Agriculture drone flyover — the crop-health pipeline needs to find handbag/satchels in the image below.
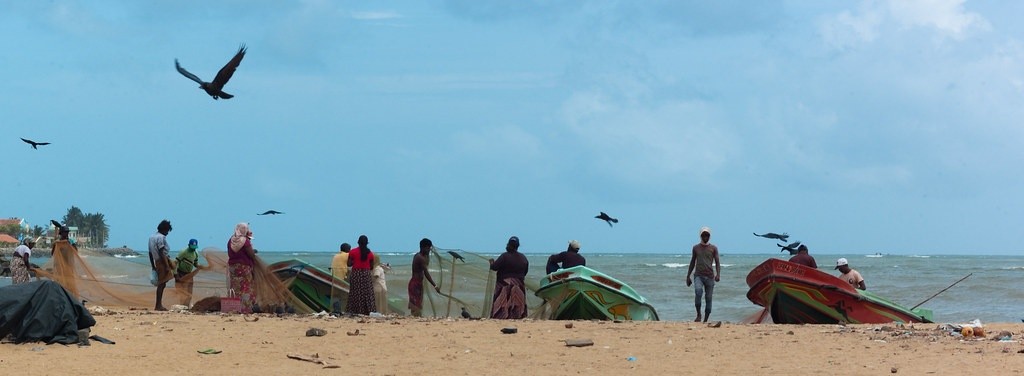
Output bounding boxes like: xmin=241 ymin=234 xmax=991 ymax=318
xmin=168 ymin=257 xmax=176 ymax=269
xmin=220 ymin=289 xmax=240 ymax=313
xmin=151 ymin=270 xmax=158 ymax=285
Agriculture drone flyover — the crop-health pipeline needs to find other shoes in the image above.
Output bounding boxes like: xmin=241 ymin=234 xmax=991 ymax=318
xmin=694 ymin=314 xmax=702 ymax=322
xmin=703 ymin=317 xmax=709 ymax=322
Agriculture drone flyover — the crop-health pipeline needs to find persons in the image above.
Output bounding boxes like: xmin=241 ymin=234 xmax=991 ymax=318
xmin=173 ymin=239 xmax=204 ymax=308
xmin=489 ymin=236 xmax=529 ymax=319
xmin=542 ymin=240 xmax=586 ymax=307
xmin=834 ymin=258 xmax=866 ymax=291
xmin=686 ymin=227 xmax=720 ymax=322
xmin=227 ymin=222 xmax=260 ymax=314
xmin=9 ymin=236 xmax=36 ymax=285
xmin=371 ymin=254 xmax=388 ymax=315
xmin=789 ymin=244 xmax=817 ymax=269
xmin=344 ymin=234 xmax=377 ymax=316
xmin=148 ymin=220 xmax=177 ymax=311
xmin=52 ymin=226 xmax=79 ymax=295
xmin=330 ymin=243 xmax=351 ymax=313
xmin=407 ymin=238 xmax=440 ymax=316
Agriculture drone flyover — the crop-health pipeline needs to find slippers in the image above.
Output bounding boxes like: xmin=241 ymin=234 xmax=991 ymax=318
xmin=155 ymin=306 xmax=168 ymax=311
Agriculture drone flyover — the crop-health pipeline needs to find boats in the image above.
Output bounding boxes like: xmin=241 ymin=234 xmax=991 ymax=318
xmin=746 ymin=259 xmax=936 ymax=325
xmin=268 ymin=260 xmax=409 ymax=315
xmin=534 ymin=265 xmax=659 ymax=321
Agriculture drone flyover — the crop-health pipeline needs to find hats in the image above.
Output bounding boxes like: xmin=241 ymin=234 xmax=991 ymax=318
xmin=568 ymin=239 xmax=580 ymax=251
xmin=247 ymin=231 xmax=254 ymax=240
xmin=58 ymin=226 xmax=71 ymax=234
xmin=834 ymin=258 xmax=848 ymax=270
xmin=188 ymin=239 xmax=198 ymax=249
xmin=22 ymin=236 xmax=37 ymax=244
xmin=508 ymin=236 xmax=519 ymax=245
xmin=700 ymin=227 xmax=711 ymax=237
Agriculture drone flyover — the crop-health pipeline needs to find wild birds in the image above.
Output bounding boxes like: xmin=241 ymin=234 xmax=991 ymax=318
xmin=174 ymin=42 xmax=249 ymax=100
xmin=753 ymin=231 xmax=801 ymax=255
xmin=446 ymin=251 xmax=466 ymax=264
xmin=461 ymin=307 xmax=470 ymax=318
xmin=595 ymin=211 xmax=618 ymax=228
xmin=20 ymin=137 xmax=52 ymax=151
xmin=920 ymin=315 xmax=934 ymax=323
xmin=256 ymin=210 xmax=285 ymax=215
